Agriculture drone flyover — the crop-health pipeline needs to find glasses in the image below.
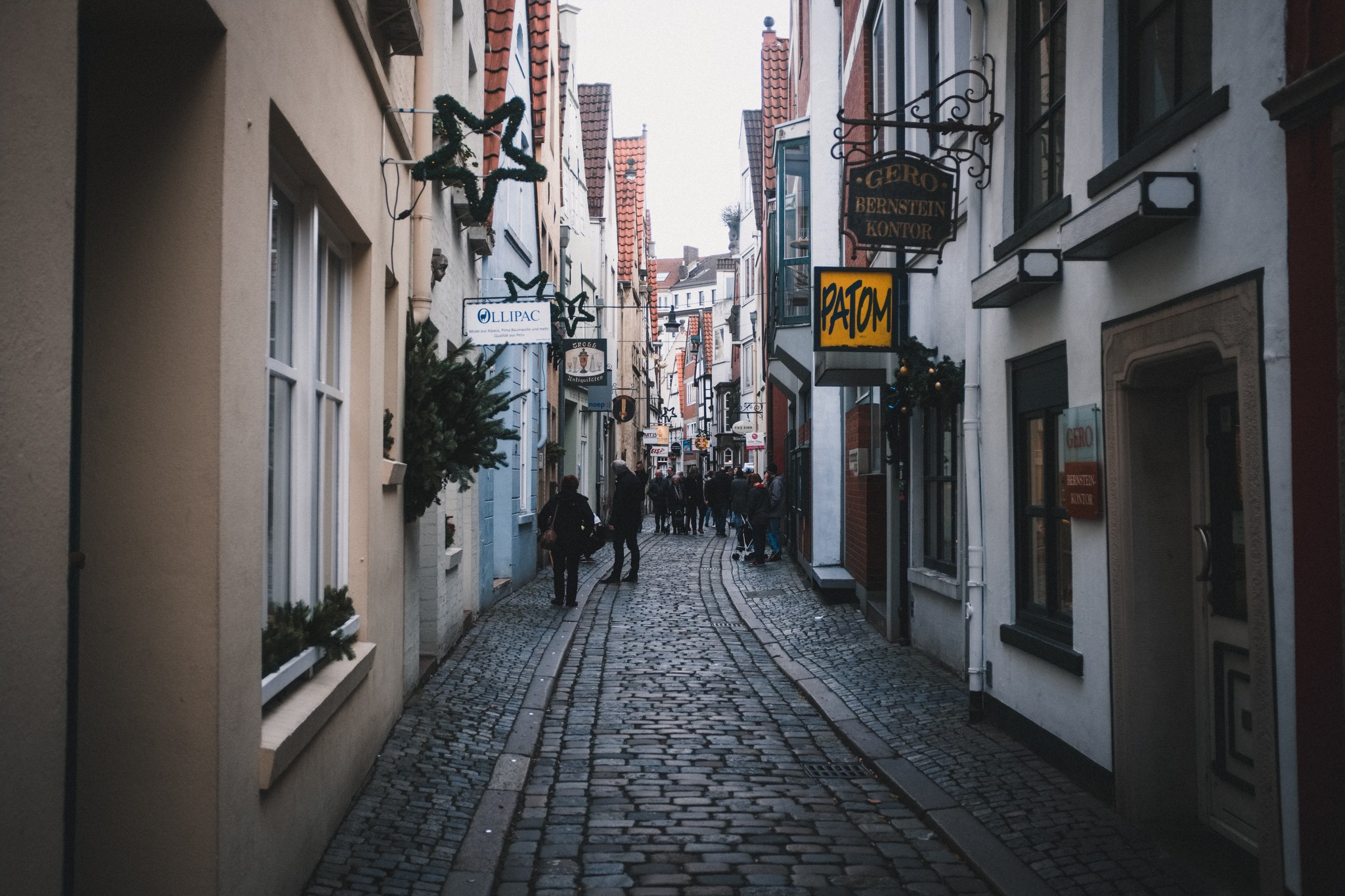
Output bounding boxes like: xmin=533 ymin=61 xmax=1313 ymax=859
xmin=667 ymin=470 xmax=672 ymax=471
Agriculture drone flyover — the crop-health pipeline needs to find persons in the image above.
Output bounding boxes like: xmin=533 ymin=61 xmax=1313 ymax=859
xmin=686 ymin=466 xmax=705 ymax=535
xmin=665 ymin=464 xmax=752 ymax=526
xmin=666 ymin=475 xmax=689 ymax=534
xmin=766 ymin=463 xmax=788 ymax=562
xmin=731 ymin=469 xmax=749 ymax=534
xmin=647 ymin=469 xmax=671 ymax=532
xmin=539 ymin=475 xmax=594 ymax=606
xmin=707 ymin=466 xmax=731 ymax=537
xmin=631 ymin=461 xmax=649 ymax=532
xmin=598 ymin=459 xmax=644 ymax=584
xmin=580 ymin=513 xmax=602 ymax=562
xmin=748 ymin=473 xmax=771 ymax=566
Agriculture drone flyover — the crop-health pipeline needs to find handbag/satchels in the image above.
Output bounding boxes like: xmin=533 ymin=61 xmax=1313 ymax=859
xmin=537 ymin=527 xmax=558 ymax=550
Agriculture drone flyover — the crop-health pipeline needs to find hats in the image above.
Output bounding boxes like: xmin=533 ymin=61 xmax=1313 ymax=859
xmin=768 ymin=464 xmax=778 ymax=474
xmin=748 ymin=473 xmax=763 ymax=486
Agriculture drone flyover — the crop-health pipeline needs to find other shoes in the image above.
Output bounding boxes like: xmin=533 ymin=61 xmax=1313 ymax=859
xmin=622 ymin=575 xmax=638 ymax=581
xmin=566 ymin=601 xmax=578 ymax=606
xmin=636 ymin=512 xmax=741 ymax=537
xmin=748 ymin=561 xmax=765 ymax=567
xmin=599 ymin=574 xmax=620 ymax=584
xmin=769 ymin=550 xmax=781 ymax=562
xmin=578 ymin=557 xmax=596 ymax=562
xmin=551 ymin=599 xmax=563 ymax=605
